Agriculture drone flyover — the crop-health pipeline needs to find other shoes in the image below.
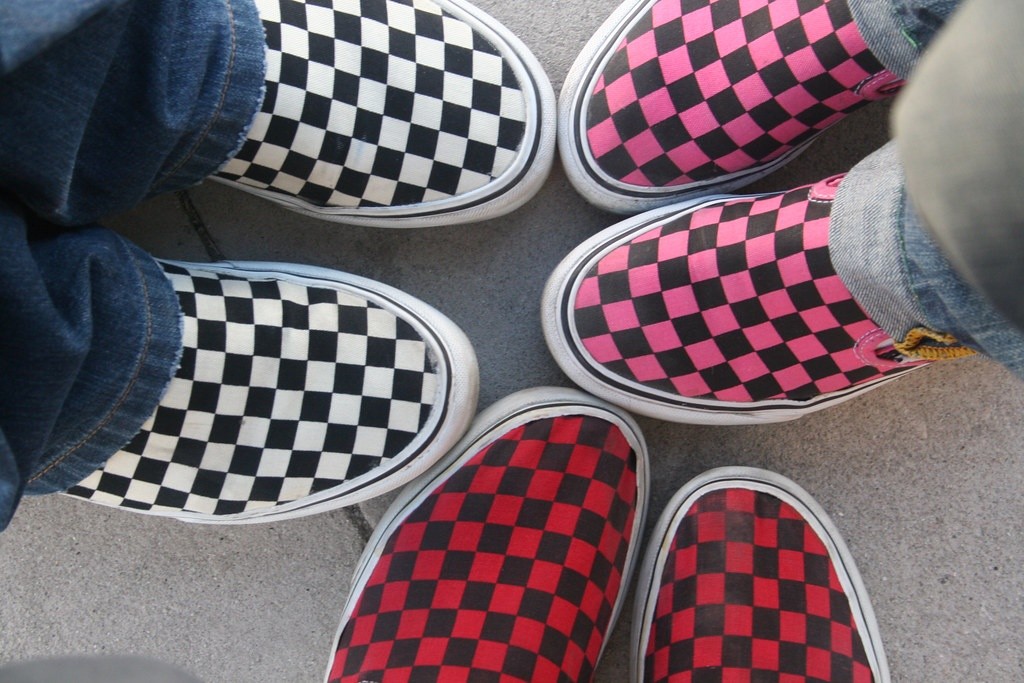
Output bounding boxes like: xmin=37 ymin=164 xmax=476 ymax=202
xmin=628 ymin=467 xmax=891 ymax=683
xmin=556 ymin=0 xmax=906 ymax=215
xmin=203 ymin=0 xmax=556 ymax=229
xmin=52 ymin=260 xmax=480 ymax=524
xmin=539 ymin=172 xmax=941 ymax=426
xmin=319 ymin=386 xmax=651 ymax=683
